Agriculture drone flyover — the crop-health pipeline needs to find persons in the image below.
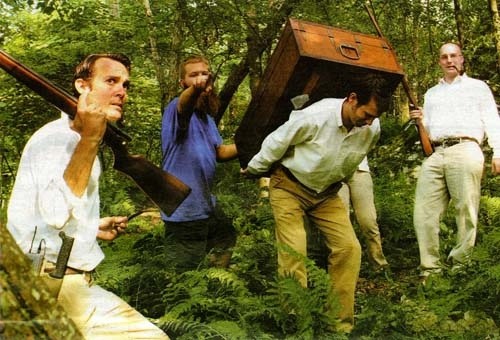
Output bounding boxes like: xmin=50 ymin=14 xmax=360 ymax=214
xmin=337 ymin=157 xmax=387 ymax=271
xmin=240 ymin=75 xmax=381 ymax=340
xmin=161 ymin=57 xmax=239 ymax=275
xmin=409 ymin=43 xmax=500 ymax=291
xmin=7 ymin=53 xmax=168 ymax=339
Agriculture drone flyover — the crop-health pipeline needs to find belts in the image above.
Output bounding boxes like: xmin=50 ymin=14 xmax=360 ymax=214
xmin=44 ymin=267 xmax=82 ymax=275
xmin=433 ymin=138 xmax=466 ymax=146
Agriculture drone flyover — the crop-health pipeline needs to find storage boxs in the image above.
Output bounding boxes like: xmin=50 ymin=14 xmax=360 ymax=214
xmin=232 ymin=17 xmax=406 ymax=178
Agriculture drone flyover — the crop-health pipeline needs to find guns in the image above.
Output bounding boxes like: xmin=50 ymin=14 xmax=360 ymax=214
xmin=0 ymin=50 xmax=191 ymax=218
xmin=363 ymin=1 xmax=434 ymax=157
